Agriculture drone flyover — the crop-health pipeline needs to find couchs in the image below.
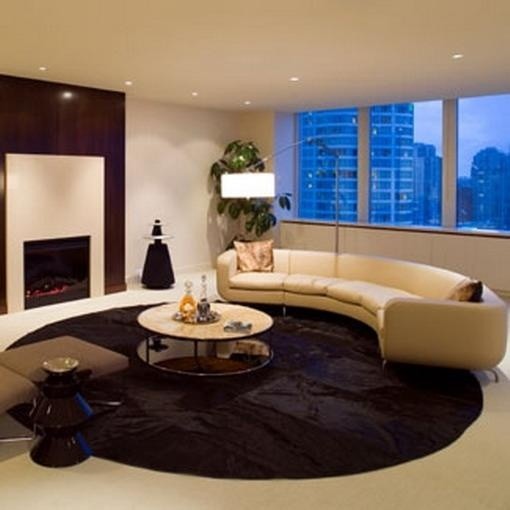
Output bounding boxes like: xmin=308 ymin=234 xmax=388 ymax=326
xmin=215 ymin=246 xmax=509 ymax=383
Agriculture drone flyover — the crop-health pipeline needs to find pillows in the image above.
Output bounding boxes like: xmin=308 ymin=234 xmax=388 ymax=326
xmin=234 ymin=238 xmax=274 ymax=270
xmin=442 ymin=277 xmax=483 ymax=304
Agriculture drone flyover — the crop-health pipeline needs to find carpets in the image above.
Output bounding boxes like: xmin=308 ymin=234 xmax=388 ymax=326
xmin=2 ymin=302 xmax=483 ymax=482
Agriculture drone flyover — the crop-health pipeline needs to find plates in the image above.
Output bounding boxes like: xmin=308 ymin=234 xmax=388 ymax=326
xmin=172 ymin=308 xmax=223 ymax=324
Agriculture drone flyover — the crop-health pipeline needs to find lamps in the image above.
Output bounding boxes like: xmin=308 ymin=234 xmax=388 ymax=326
xmin=220 ymin=136 xmax=340 ymax=252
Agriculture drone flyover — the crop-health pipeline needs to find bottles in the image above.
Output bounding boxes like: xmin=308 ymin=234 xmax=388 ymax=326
xmin=179 ymin=274 xmax=210 ymax=324
